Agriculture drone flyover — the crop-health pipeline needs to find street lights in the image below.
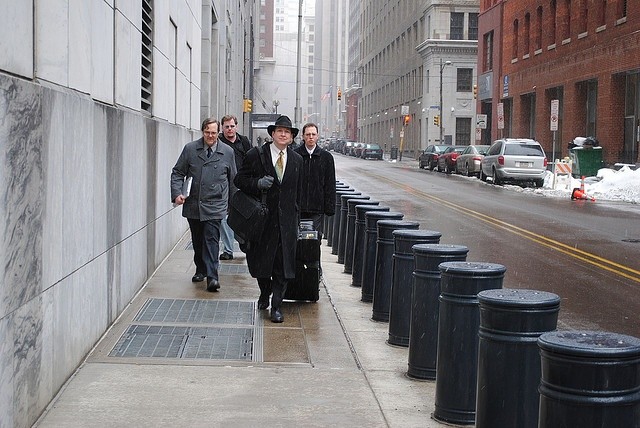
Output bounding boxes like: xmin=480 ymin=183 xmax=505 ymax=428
xmin=439 ymin=59 xmax=451 ymax=144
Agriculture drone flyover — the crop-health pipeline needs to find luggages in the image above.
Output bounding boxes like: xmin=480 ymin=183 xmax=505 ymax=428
xmin=283 ymin=205 xmax=320 ymax=301
xmin=297 ymin=230 xmax=320 ymax=262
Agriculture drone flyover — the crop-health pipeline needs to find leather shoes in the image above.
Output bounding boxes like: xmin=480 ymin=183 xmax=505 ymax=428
xmin=193 ymin=272 xmax=204 ymax=282
xmin=258 ymin=289 xmax=272 ymax=309
xmin=207 ymin=277 xmax=220 ymax=289
xmin=270 ymin=307 xmax=284 ymax=323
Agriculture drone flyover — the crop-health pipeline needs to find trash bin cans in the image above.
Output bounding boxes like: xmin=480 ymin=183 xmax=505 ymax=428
xmin=571 ymin=146 xmax=603 ymax=179
xmin=566 ymin=149 xmax=575 ymax=159
xmin=390 ymin=147 xmax=398 ymax=159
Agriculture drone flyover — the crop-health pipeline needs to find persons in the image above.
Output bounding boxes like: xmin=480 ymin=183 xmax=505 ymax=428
xmin=168 ymin=117 xmax=238 ymax=290
xmin=217 ymin=114 xmax=253 ymax=260
xmin=234 ymin=116 xmax=305 ymax=322
xmin=295 ymin=123 xmax=335 ymax=282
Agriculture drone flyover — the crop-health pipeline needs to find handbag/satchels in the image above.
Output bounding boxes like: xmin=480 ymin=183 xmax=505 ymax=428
xmin=298 ymin=221 xmax=313 ymax=233
xmin=227 ymin=190 xmax=270 ymax=241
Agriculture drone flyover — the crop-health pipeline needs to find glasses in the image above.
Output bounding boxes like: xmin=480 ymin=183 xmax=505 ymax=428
xmin=225 ymin=125 xmax=235 ymax=128
xmin=204 ymin=131 xmax=218 ymax=137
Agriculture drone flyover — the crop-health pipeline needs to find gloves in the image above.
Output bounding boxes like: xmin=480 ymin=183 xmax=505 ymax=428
xmin=257 ymin=175 xmax=274 ymax=189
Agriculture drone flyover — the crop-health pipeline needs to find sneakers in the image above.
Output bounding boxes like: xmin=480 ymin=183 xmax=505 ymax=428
xmin=220 ymin=252 xmax=233 ymax=260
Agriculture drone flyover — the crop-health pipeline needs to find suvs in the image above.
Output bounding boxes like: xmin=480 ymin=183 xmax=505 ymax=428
xmin=480 ymin=138 xmax=547 ymax=186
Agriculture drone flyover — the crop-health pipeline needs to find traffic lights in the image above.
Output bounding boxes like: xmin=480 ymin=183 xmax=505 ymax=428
xmin=474 ymin=85 xmax=477 ymax=98
xmin=243 ymin=100 xmax=252 ymax=112
xmin=434 ymin=116 xmax=440 ymax=125
xmin=338 ymin=89 xmax=341 ymax=100
xmin=403 ymin=113 xmax=411 ymax=126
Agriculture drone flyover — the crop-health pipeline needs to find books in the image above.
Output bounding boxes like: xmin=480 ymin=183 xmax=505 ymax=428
xmin=172 ymin=177 xmax=193 ymax=208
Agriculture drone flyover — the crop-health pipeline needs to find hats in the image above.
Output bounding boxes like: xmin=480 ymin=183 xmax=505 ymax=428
xmin=267 ymin=116 xmax=299 ymax=138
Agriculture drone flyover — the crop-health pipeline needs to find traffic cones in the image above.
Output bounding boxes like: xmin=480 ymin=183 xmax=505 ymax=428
xmin=581 ymin=176 xmax=585 ymax=193
xmin=571 ymin=188 xmax=595 ymax=201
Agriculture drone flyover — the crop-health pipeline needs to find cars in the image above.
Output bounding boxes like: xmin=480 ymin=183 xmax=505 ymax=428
xmin=456 ymin=144 xmax=490 ymax=177
xmin=420 ymin=144 xmax=452 ymax=170
xmin=361 ymin=143 xmax=383 ymax=159
xmin=354 ymin=143 xmax=365 ymax=157
xmin=436 ymin=145 xmax=467 ymax=173
xmin=342 ymin=142 xmax=352 ymax=155
xmin=325 ymin=138 xmax=343 ymax=152
xmin=349 ymin=142 xmax=356 ymax=155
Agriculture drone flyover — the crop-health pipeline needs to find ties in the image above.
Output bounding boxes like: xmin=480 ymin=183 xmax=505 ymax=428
xmin=207 ymin=147 xmax=212 ymax=158
xmin=274 ymin=151 xmax=284 ymax=184
